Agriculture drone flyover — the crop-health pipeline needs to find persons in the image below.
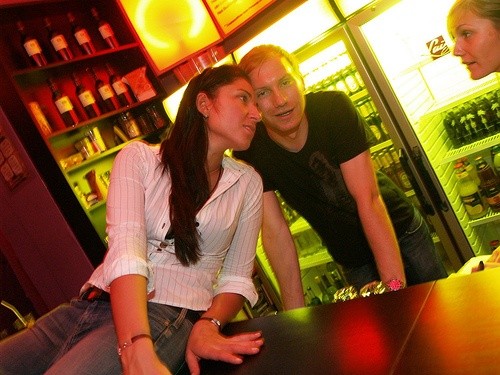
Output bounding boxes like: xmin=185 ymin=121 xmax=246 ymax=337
xmin=447 ymin=0 xmax=500 ymax=273
xmin=0 ymin=65 xmax=264 ymax=375
xmin=231 ymin=45 xmax=448 ymax=313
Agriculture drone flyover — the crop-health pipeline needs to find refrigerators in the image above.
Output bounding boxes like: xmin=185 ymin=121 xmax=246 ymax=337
xmin=224 ymin=0 xmax=500 ymax=309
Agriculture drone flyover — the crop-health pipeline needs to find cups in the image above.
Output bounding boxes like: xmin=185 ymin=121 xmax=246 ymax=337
xmin=86 ymin=126 xmax=106 ymax=152
xmin=75 ymin=135 xmax=100 ymax=160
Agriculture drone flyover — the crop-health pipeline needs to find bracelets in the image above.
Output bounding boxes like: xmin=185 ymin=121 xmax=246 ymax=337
xmin=116 ymin=332 xmax=152 ymax=357
xmin=198 ymin=316 xmax=222 ymax=330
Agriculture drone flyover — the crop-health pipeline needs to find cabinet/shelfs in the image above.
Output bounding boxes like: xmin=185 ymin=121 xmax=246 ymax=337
xmin=0 ymin=0 xmax=171 ymax=252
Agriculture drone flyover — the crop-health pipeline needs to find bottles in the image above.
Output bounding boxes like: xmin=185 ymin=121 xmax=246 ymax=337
xmin=443 ymin=88 xmax=500 ymax=144
xmin=274 ymin=190 xmax=302 ymax=227
xmin=68 ymin=11 xmax=96 ymax=55
xmin=301 ymin=261 xmax=350 ymax=306
xmin=354 ymin=96 xmax=391 ymax=143
xmin=15 ymin=21 xmax=49 ymax=67
xmin=370 ymin=146 xmax=412 ymax=192
xmin=454 ymin=145 xmax=500 ymax=219
xmin=489 ymin=239 xmax=500 ymax=252
xmin=47 ymin=62 xmax=131 ymax=129
xmin=303 ymin=51 xmax=366 ymax=97
xmin=92 ymin=7 xmax=119 ymax=49
xmin=117 ymin=103 xmax=166 ymax=139
xmin=409 ymin=194 xmax=436 ymax=233
xmin=44 ymin=13 xmax=73 ymax=61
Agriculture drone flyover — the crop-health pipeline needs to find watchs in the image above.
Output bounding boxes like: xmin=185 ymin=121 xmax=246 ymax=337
xmin=386 ymin=279 xmax=405 ymax=291
